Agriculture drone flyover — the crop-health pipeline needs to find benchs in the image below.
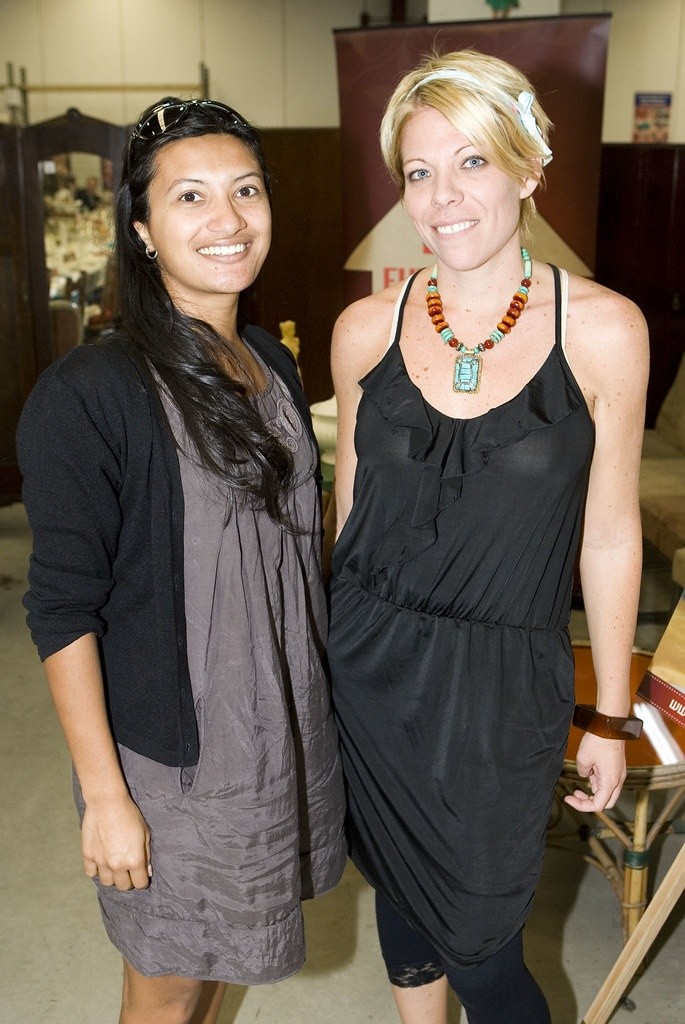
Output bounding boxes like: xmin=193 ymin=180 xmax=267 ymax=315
xmin=639 ymin=357 xmax=685 ymax=566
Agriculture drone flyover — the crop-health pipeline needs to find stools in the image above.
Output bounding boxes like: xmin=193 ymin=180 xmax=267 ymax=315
xmin=544 ymin=639 xmax=685 ymax=977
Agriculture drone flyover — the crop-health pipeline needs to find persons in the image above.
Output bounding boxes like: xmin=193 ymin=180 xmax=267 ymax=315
xmin=16 ymin=95 xmax=348 ymax=1024
xmin=55 ymin=176 xmax=103 ymax=212
xmin=319 ymin=49 xmax=651 ymax=1024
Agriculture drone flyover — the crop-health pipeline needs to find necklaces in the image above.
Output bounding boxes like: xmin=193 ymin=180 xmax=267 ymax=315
xmin=425 ymin=246 xmax=532 ymax=393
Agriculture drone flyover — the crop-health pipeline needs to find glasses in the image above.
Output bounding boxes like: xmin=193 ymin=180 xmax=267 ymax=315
xmin=127 ymin=100 xmax=251 ymax=180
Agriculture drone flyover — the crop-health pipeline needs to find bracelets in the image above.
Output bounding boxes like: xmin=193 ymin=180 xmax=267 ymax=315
xmin=571 ymin=704 xmax=644 ymax=741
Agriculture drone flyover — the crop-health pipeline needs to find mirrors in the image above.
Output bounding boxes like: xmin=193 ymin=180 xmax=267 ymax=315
xmin=36 ymin=148 xmax=119 ymax=371
